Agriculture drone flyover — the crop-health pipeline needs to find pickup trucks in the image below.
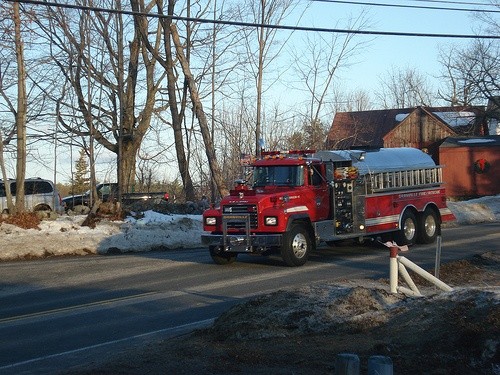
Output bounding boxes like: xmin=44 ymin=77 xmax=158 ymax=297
xmin=62 ymin=182 xmax=169 ymax=209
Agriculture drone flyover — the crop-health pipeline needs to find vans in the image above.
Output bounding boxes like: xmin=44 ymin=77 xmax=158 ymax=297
xmin=0 ymin=177 xmax=62 ymax=214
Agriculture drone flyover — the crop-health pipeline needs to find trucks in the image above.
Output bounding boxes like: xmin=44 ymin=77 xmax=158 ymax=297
xmin=198 ymin=146 xmax=457 ymax=267
